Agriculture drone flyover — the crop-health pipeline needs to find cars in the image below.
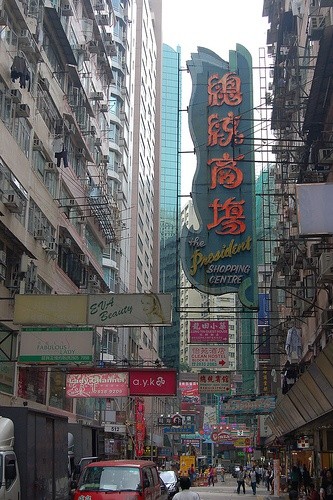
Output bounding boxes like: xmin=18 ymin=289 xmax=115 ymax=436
xmin=157 ymin=471 xmax=180 ymax=496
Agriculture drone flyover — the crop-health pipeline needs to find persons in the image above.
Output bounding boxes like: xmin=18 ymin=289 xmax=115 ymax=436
xmin=190 ymin=463 xmax=333 ymax=500
xmin=172 ymin=476 xmax=200 ymax=500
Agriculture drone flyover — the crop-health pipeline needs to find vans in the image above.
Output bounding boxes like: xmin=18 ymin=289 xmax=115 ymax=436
xmin=0 ymin=416 xmax=21 ymax=500
xmin=70 ymin=460 xmax=161 ymax=500
